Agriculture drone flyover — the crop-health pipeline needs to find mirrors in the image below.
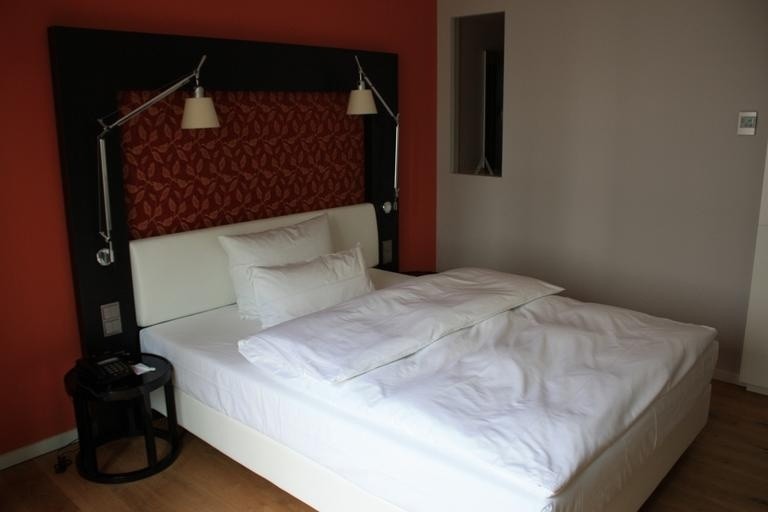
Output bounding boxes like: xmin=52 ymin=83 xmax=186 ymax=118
xmin=453 ymin=12 xmax=504 ymax=178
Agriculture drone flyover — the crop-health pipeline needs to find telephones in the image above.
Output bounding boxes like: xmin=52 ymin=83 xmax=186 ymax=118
xmin=76 ymin=351 xmax=134 ymax=386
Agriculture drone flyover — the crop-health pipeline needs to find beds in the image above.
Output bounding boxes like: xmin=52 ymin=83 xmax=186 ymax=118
xmin=127 ymin=202 xmax=720 ymax=512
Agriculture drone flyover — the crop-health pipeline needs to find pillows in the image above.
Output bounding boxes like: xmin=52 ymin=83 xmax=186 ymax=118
xmin=218 ymin=212 xmax=334 ymax=321
xmin=248 ymin=241 xmax=376 ymax=331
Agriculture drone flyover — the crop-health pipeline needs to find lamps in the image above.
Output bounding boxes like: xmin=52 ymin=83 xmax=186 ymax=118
xmin=346 ymin=52 xmax=400 ymax=271
xmin=96 ymin=56 xmax=221 ymax=265
